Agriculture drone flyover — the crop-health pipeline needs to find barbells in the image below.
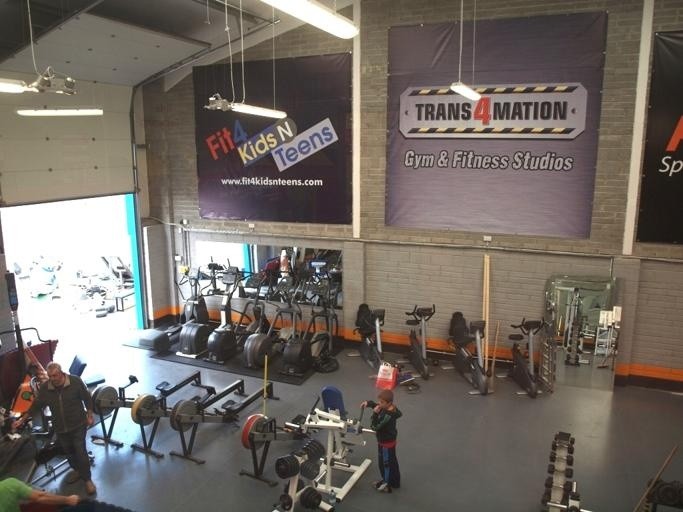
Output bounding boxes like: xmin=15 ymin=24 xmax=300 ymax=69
xmin=542 ymin=434 xmax=575 ymax=507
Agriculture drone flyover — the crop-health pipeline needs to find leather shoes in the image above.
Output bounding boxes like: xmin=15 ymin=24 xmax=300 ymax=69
xmin=67 ymin=471 xmax=96 ymax=494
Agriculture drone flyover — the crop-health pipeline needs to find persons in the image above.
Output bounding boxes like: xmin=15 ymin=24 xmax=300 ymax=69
xmin=360 ymin=389 xmax=402 ymax=492
xmin=0 ymin=477 xmax=80 ymax=512
xmin=13 ymin=363 xmax=96 ymax=494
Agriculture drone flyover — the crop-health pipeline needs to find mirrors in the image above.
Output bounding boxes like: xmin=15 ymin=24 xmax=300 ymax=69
xmin=545 ymin=273 xmax=625 ymax=396
xmin=194 ymin=240 xmax=344 ymax=310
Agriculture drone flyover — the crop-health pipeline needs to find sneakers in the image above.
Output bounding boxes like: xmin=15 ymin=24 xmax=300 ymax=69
xmin=376 ymin=479 xmax=387 ymax=490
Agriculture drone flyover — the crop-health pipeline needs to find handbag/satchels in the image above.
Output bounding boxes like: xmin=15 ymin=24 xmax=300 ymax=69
xmin=376 ymin=365 xmax=397 ymax=390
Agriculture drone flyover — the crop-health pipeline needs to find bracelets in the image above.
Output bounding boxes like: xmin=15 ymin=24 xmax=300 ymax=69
xmin=18 ymin=418 xmax=24 ymax=422
